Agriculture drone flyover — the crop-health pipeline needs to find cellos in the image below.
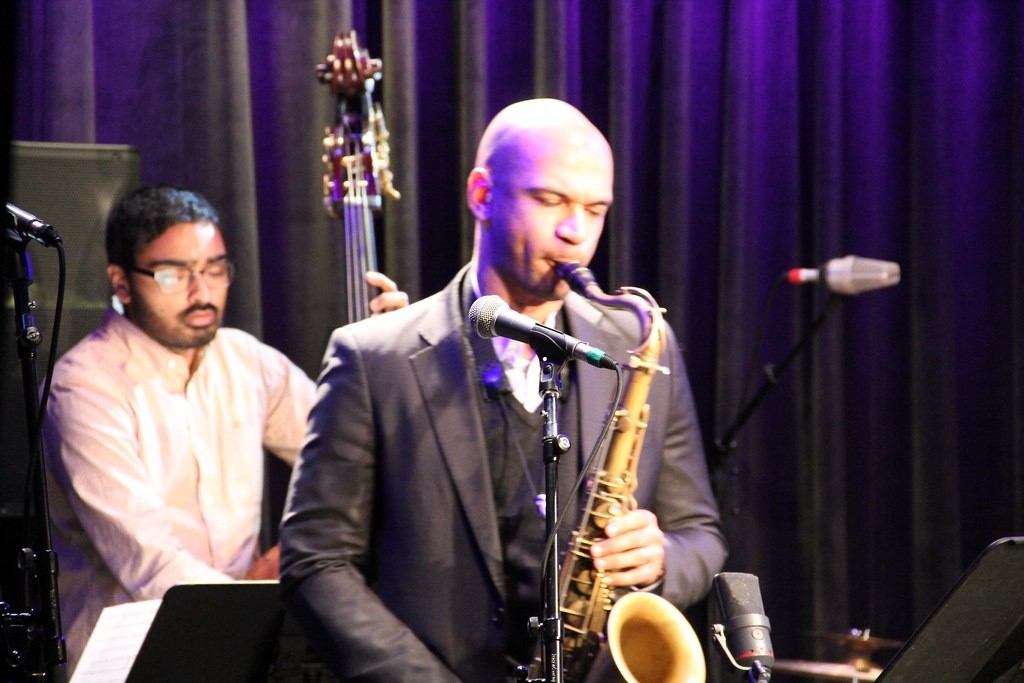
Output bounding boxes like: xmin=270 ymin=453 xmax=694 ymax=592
xmin=308 ymin=25 xmax=404 ymax=327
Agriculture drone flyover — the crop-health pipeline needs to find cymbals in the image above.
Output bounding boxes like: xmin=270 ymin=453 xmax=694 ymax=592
xmin=804 ymin=626 xmax=905 ymax=653
xmin=767 ymin=656 xmax=885 ymax=681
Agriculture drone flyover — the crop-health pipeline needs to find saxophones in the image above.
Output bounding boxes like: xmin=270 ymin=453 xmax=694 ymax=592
xmin=510 ymin=258 xmax=711 ymax=683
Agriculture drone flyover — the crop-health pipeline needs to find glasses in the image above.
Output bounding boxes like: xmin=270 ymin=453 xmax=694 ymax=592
xmin=129 ymin=260 xmax=235 ymax=295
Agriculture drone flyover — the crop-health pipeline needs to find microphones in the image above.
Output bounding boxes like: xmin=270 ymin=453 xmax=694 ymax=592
xmin=782 ymin=255 xmax=901 ymax=295
xmin=7 ymin=202 xmax=63 ymax=248
xmin=468 ymin=295 xmax=619 ymax=370
xmin=713 ymin=572 xmax=775 ymax=683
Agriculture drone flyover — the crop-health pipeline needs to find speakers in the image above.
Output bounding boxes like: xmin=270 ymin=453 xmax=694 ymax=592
xmin=0 ymin=139 xmax=143 ymax=515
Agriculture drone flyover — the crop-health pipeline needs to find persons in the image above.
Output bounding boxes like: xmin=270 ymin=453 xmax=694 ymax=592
xmin=38 ymin=183 xmax=410 ymax=683
xmin=275 ymin=96 xmax=731 ymax=683
